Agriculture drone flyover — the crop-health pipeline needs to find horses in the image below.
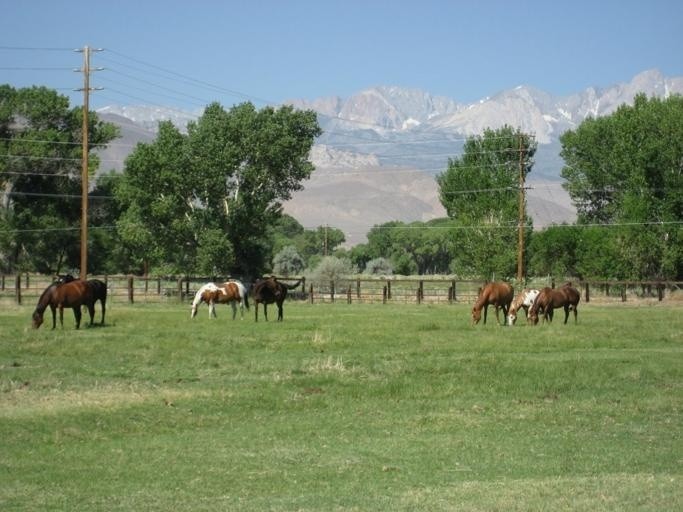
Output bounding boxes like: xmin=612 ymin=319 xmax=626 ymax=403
xmin=252 ymin=276 xmax=302 ymax=323
xmin=508 ymin=286 xmax=580 ymax=327
xmin=472 ymin=282 xmax=514 ymax=325
xmin=31 ymin=274 xmax=107 ymax=331
xmin=190 ymin=281 xmax=250 ymax=321
